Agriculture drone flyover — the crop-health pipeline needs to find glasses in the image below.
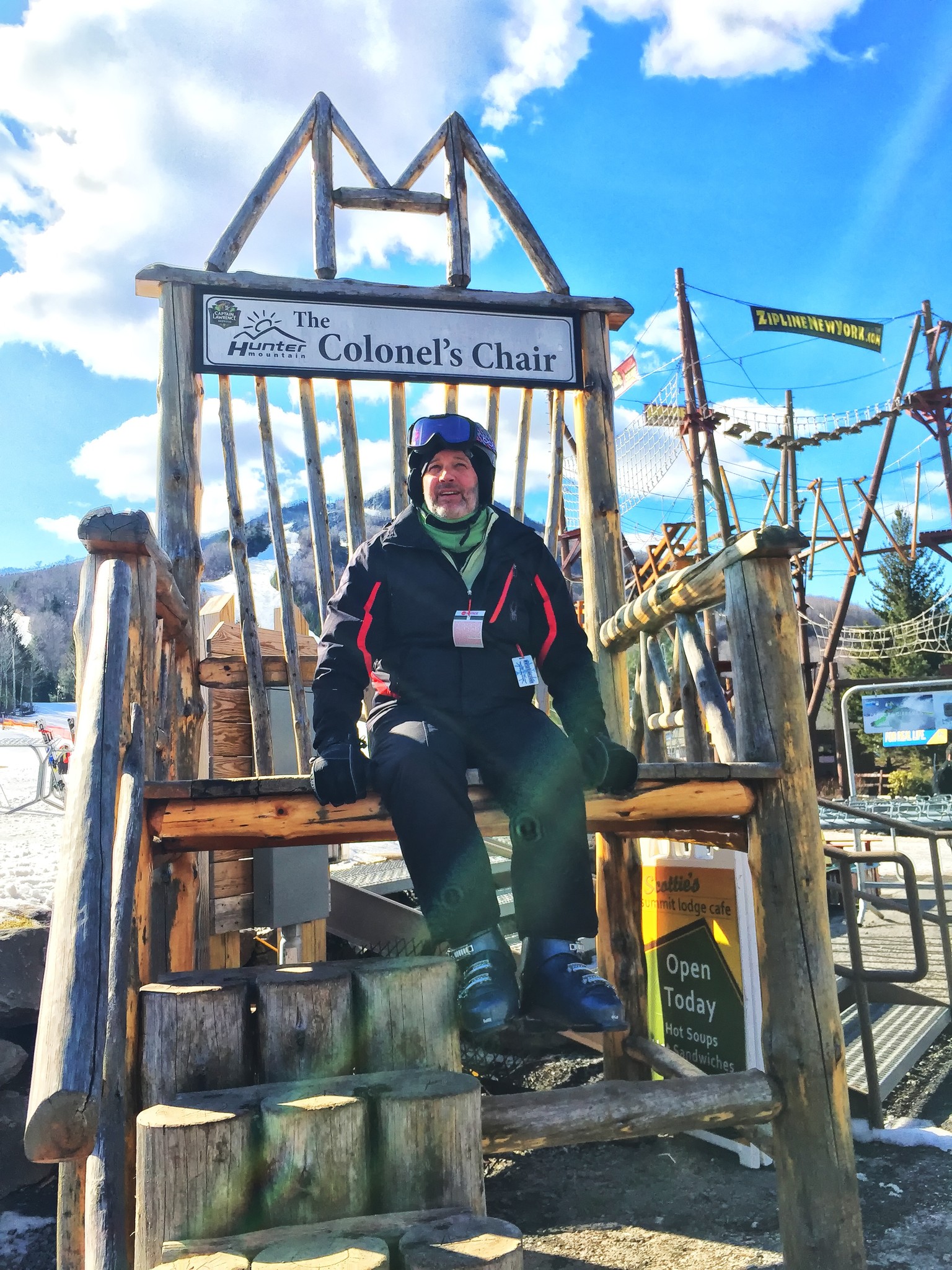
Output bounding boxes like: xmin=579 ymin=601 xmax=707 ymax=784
xmin=406 ymin=416 xmax=498 ymax=456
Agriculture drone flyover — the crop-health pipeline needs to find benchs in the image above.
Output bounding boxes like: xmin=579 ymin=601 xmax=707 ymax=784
xmin=148 ymin=741 xmax=762 ymax=877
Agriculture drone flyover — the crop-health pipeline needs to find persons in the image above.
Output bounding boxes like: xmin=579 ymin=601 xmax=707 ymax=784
xmin=308 ymin=413 xmax=637 ymax=1032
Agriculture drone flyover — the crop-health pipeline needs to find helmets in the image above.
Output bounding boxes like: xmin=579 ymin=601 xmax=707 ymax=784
xmin=407 ymin=413 xmax=498 ymax=511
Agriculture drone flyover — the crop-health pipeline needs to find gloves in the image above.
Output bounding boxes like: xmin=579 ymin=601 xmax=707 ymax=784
xmin=579 ymin=730 xmax=639 ymax=796
xmin=309 ymin=731 xmax=378 ymax=808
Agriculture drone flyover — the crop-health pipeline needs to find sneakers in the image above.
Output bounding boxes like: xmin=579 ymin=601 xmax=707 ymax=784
xmin=518 ymin=935 xmax=629 ymax=1034
xmin=445 ymin=922 xmax=521 ymax=1033
